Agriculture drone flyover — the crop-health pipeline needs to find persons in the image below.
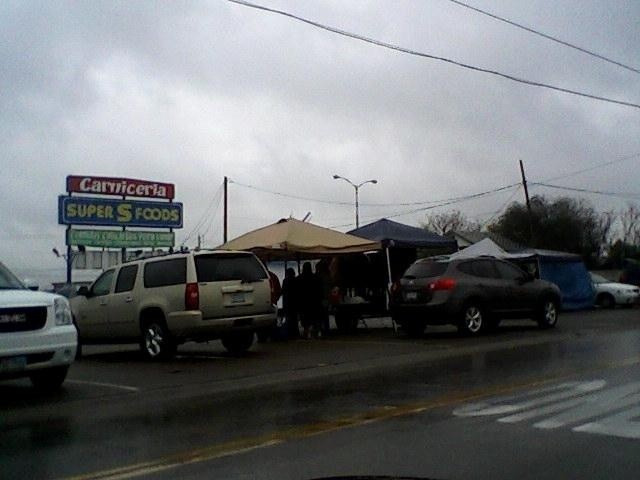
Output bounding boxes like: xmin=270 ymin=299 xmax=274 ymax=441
xmin=312 ymin=258 xmax=336 ymax=340
xmin=292 ymin=261 xmax=316 ymax=332
xmin=280 ymin=265 xmax=302 ymax=336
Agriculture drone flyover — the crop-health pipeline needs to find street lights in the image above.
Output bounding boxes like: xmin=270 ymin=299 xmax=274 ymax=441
xmin=333 ymin=175 xmax=377 ymax=229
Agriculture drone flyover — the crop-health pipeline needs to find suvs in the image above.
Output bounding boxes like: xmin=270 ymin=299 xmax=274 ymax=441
xmin=387 ymin=255 xmax=562 ymax=338
xmin=71 ymin=249 xmax=279 ymax=362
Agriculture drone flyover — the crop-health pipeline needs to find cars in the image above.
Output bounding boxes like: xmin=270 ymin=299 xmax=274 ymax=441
xmin=0 ymin=261 xmax=79 ymax=393
xmin=588 ymin=272 xmax=640 ymax=310
